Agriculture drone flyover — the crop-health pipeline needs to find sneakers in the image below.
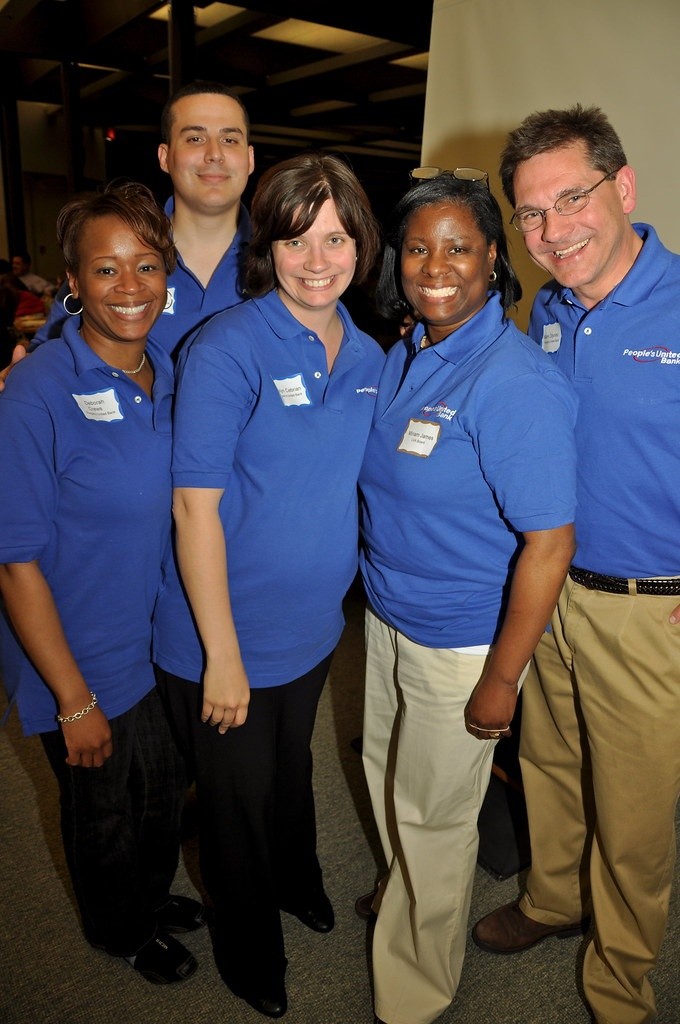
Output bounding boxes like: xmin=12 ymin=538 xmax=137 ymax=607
xmin=471 ymin=903 xmax=591 ymax=954
xmin=125 ymin=892 xmax=207 ymax=986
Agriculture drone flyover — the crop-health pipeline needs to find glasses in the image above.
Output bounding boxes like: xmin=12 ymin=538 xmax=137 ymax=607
xmin=405 ymin=166 xmax=491 ymax=194
xmin=509 ymin=165 xmax=622 ymax=233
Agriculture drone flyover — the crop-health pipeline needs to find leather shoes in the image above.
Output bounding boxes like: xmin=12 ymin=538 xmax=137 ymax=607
xmin=243 ymin=980 xmax=289 ymax=1018
xmin=294 ymin=893 xmax=335 ymax=933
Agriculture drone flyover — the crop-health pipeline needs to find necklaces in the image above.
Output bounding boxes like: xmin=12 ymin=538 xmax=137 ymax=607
xmin=421 ymin=335 xmax=427 ymax=349
xmin=121 ymin=353 xmax=146 ymax=374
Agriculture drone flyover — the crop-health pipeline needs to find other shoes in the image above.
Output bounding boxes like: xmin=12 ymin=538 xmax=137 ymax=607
xmin=355 ymin=890 xmax=380 ymax=920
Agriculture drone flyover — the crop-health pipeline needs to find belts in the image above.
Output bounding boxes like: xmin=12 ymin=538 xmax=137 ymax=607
xmin=568 ymin=565 xmax=680 ymax=595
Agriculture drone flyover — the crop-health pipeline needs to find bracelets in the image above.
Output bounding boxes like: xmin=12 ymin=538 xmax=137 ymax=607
xmin=57 ymin=690 xmax=97 ymax=723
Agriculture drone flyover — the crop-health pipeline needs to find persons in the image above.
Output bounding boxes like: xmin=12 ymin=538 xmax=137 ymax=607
xmin=0 ymin=79 xmax=680 ymax=1024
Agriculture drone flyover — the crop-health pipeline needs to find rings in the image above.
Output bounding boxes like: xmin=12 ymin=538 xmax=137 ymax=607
xmin=489 ymin=732 xmax=500 ymax=739
